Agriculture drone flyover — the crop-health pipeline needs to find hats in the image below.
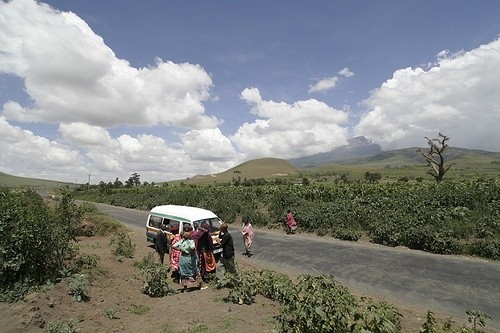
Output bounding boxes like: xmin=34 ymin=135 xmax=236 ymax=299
xmin=203 ymin=224 xmax=210 ymax=229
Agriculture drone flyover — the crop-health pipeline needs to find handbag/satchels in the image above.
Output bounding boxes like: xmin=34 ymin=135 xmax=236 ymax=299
xmin=171 ymin=240 xmax=184 ymax=250
xmin=180 ymin=239 xmax=190 ymax=254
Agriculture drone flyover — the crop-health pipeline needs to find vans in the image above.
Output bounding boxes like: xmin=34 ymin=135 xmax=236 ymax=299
xmin=145 ymin=205 xmax=234 ymax=256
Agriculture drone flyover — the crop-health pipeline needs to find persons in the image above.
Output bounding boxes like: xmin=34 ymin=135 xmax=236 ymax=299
xmin=155 ymin=224 xmax=167 ymax=265
xmin=219 ymin=224 xmax=236 ymax=274
xmin=286 ymin=210 xmax=296 ymax=234
xmin=171 ymin=232 xmax=209 ymax=294
xmin=242 ymin=216 xmax=254 ymax=257
xmin=169 ymin=223 xmax=217 ymax=283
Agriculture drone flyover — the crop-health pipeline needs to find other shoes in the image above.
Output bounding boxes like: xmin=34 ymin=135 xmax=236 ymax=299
xmin=200 ymin=286 xmax=208 ymax=290
